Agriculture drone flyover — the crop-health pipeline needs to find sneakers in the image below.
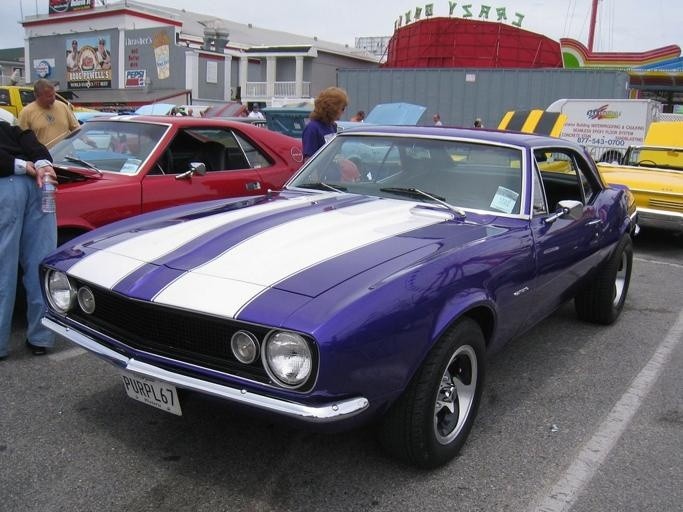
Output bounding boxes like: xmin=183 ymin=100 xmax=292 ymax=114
xmin=25 ymin=338 xmax=45 ymax=355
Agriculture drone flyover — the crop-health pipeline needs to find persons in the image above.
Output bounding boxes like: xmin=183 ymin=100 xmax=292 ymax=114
xmin=93 ymin=37 xmax=111 ymax=87
xmin=0 ymin=118 xmax=61 ymax=360
xmin=349 ymin=110 xmax=365 ymax=123
xmin=11 ymin=78 xmax=81 ymax=150
xmin=66 ymin=40 xmax=83 ymax=87
xmin=471 ymin=118 xmax=485 ymax=128
xmin=430 ymin=112 xmax=444 ymax=127
xmin=299 ymin=86 xmax=362 ymax=185
xmin=168 ymin=105 xmax=212 ymax=117
xmin=244 ymin=102 xmax=265 ymax=128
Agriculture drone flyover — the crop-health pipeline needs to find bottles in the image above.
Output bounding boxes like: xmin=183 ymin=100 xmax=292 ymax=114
xmin=42 ymin=172 xmax=57 ymax=214
